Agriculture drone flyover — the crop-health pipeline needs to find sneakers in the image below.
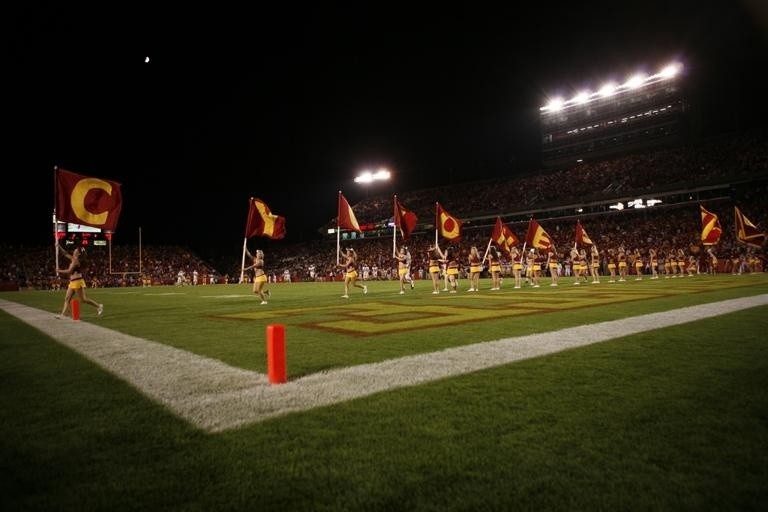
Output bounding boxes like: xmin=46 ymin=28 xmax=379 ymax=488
xmin=341 ymin=294 xmax=348 ymax=299
xmin=634 ymin=275 xmax=694 ymax=281
xmin=260 ymin=302 xmax=267 ymax=306
xmin=574 ymin=278 xmax=627 ymax=284
xmin=54 ymin=316 xmax=69 ymax=320
xmin=363 ymin=287 xmax=367 ymax=296
xmin=97 ymin=304 xmax=104 ymax=316
xmin=514 ymin=282 xmax=557 ymax=289
xmin=266 ymin=289 xmax=271 ymax=297
xmin=433 ymin=287 xmax=501 ymax=295
xmin=399 ymin=280 xmax=414 ymax=295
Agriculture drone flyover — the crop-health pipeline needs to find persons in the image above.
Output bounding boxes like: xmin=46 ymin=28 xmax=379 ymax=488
xmin=54 ymin=244 xmax=103 ymax=319
xmin=2 ymin=238 xmax=230 ymax=292
xmin=223 ymin=130 xmax=767 ymax=282
xmin=242 ymin=243 xmax=589 ymax=304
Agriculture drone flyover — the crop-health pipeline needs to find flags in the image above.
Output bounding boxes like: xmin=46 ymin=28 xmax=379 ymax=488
xmin=54 ymin=167 xmax=122 ymax=231
